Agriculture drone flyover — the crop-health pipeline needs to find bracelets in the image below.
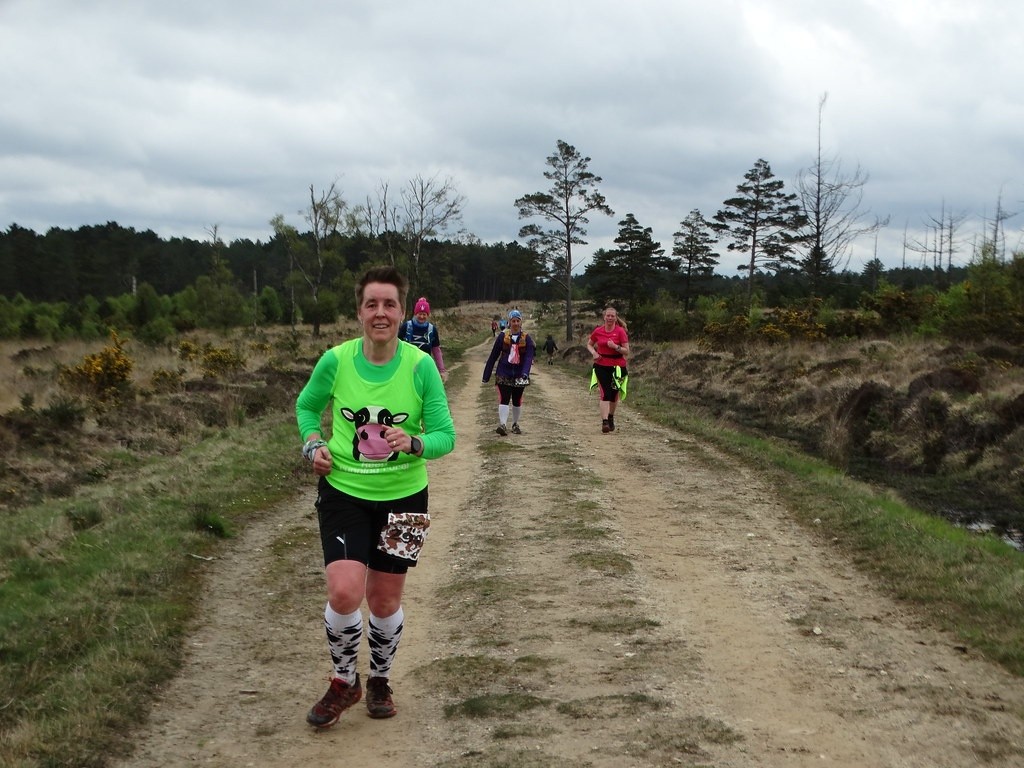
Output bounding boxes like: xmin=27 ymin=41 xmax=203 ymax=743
xmin=616 ymin=345 xmax=621 ymax=352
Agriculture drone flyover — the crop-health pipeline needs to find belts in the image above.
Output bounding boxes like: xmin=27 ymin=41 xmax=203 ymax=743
xmin=598 ymin=353 xmax=624 ymax=359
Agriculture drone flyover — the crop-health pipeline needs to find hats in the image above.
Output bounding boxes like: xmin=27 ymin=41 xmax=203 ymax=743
xmin=509 ymin=310 xmax=521 ymax=320
xmin=414 ymin=297 xmax=430 ymax=316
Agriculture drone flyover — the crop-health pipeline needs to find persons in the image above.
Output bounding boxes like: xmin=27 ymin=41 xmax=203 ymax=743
xmin=296 ymin=266 xmax=456 ymax=729
xmin=543 ymin=335 xmax=558 ymax=365
xmin=482 ymin=310 xmax=536 ymax=436
xmin=586 ymin=307 xmax=628 ymax=433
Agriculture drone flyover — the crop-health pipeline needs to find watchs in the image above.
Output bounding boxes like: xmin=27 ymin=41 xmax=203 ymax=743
xmin=406 ymin=436 xmax=421 ymax=455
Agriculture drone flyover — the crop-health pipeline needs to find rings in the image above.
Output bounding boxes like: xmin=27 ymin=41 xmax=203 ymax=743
xmin=393 ymin=440 xmax=396 ymax=447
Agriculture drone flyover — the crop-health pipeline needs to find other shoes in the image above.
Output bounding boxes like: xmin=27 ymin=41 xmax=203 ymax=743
xmin=548 ymin=361 xmax=554 ymax=365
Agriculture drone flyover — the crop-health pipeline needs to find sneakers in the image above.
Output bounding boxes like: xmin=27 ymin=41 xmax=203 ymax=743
xmin=602 ymin=423 xmax=610 ymax=433
xmin=512 ymin=423 xmax=521 ymax=433
xmin=366 ymin=675 xmax=397 ymax=718
xmin=306 ymin=672 xmax=362 ymax=727
xmin=608 ymin=417 xmax=614 ymax=431
xmin=496 ymin=424 xmax=508 ymax=436
